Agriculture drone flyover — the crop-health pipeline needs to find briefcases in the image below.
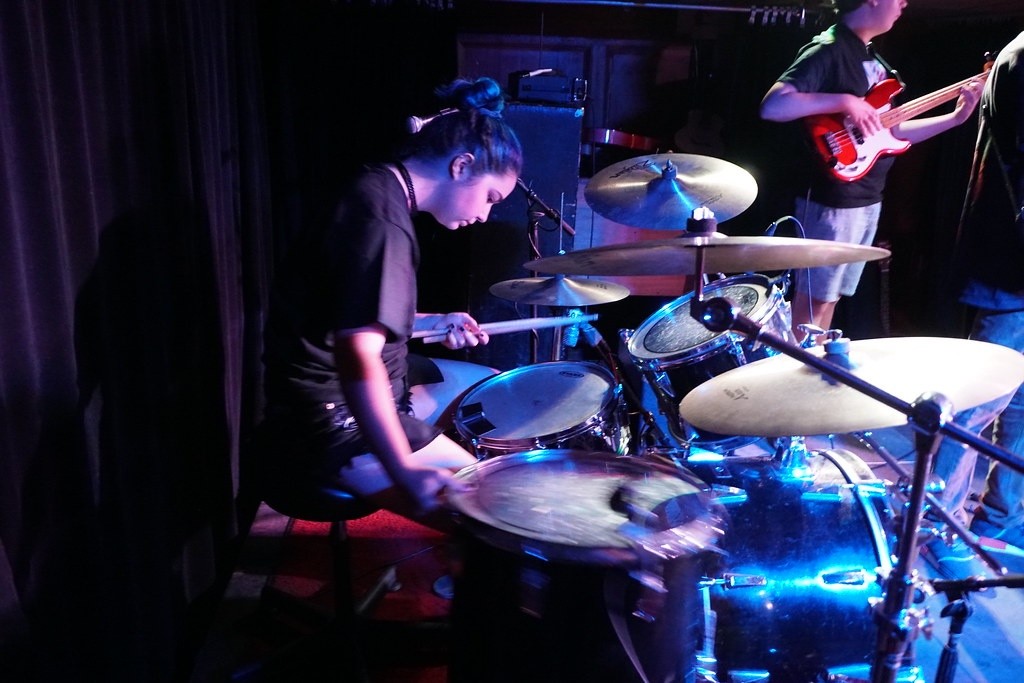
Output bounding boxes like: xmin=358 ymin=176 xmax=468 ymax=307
xmin=507 ymin=67 xmax=589 ymax=106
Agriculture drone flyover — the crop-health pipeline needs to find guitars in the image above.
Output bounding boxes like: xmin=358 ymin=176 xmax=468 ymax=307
xmin=801 ymin=51 xmax=998 ymax=188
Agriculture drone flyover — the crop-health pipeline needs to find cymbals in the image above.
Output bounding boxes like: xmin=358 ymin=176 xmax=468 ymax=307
xmin=487 ymin=273 xmax=632 ymax=308
xmin=676 ymin=333 xmax=1024 ymax=438
xmin=522 ymin=216 xmax=895 ymax=279
xmin=583 ymin=151 xmax=759 ymax=233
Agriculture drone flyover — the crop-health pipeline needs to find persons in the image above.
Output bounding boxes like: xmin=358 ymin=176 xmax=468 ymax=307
xmin=760 ymin=0 xmax=984 ymax=344
xmin=259 ymin=78 xmax=522 ymax=526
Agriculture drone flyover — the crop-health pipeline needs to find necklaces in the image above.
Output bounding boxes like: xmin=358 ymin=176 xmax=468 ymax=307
xmin=396 ymin=160 xmax=418 ymax=213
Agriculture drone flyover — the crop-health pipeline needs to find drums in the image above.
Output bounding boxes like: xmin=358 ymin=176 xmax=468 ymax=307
xmin=625 ymin=271 xmax=805 ymax=454
xmin=436 ymin=448 xmax=729 ymax=683
xmin=667 ymin=444 xmax=920 ymax=683
xmin=453 ymin=358 xmax=631 ymax=460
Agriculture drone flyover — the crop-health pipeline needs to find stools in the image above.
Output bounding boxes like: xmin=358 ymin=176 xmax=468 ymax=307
xmin=206 ymin=398 xmax=454 ymax=683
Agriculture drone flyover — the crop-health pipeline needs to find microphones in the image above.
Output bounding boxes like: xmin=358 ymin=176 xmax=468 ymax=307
xmin=407 ymin=106 xmax=459 ymax=133
xmin=745 ymin=223 xmax=777 ymax=276
xmin=563 ymin=309 xmax=581 ymax=347
